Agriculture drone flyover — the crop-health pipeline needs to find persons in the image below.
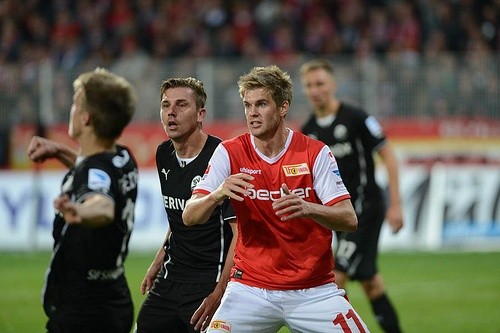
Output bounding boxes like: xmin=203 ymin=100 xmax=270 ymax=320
xmin=135 ymin=77 xmax=238 ymax=333
xmin=0 ymin=1 xmax=500 ymax=129
xmin=297 ymin=60 xmax=404 ymax=333
xmin=28 ymin=69 xmax=140 ymax=333
xmin=182 ymin=66 xmax=369 ymax=333
